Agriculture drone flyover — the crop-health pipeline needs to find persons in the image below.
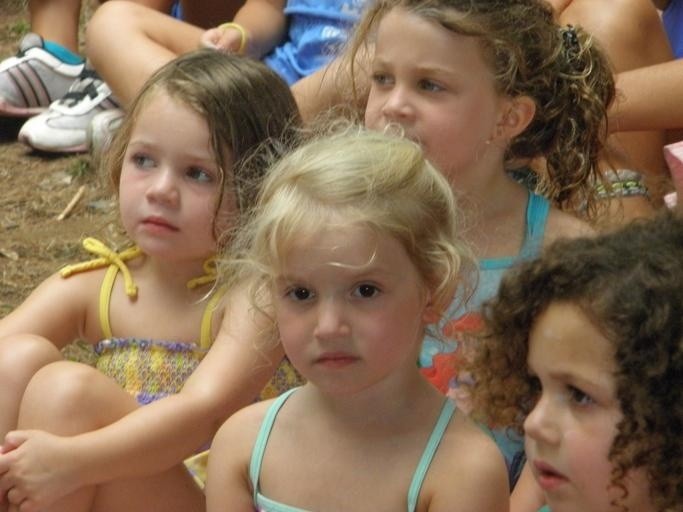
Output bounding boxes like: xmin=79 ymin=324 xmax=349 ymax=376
xmin=1 ymin=0 xmax=682 ymax=511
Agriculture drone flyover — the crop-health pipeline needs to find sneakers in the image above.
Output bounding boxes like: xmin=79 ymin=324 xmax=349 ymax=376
xmin=1 ymin=33 xmax=86 ymax=116
xmin=18 ymin=62 xmax=126 ymax=153
